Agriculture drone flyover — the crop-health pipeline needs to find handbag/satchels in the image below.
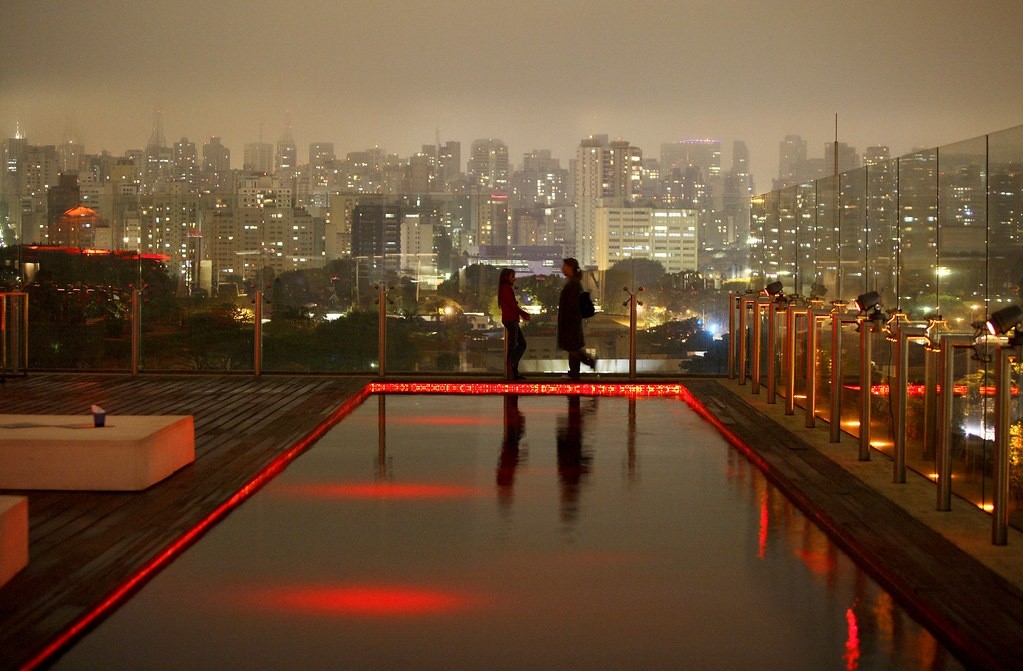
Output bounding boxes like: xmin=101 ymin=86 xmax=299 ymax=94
xmin=579 ymin=292 xmax=597 ymax=320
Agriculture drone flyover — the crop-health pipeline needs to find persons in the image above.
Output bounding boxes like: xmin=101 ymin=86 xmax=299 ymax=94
xmin=88 ymin=290 xmax=123 ymax=336
xmin=497 ymin=393 xmax=525 ymax=485
xmin=556 ymin=257 xmax=596 ymax=379
xmin=557 ymin=395 xmax=583 ymax=488
xmin=497 ymin=267 xmax=531 ymax=379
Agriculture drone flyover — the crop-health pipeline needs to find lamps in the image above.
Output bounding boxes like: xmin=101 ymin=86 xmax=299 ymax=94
xmin=986 ymin=305 xmax=1022 ymax=347
xmin=854 ymin=291 xmax=887 ymax=320
xmin=763 ymin=282 xmax=790 ymax=301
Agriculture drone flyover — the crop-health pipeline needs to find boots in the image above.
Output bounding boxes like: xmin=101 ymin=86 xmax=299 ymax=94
xmin=513 ymin=363 xmax=526 ymax=380
xmin=506 ymin=363 xmax=518 ymax=382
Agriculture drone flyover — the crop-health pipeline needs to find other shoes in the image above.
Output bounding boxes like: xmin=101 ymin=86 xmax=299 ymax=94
xmin=561 ymin=371 xmax=579 ymax=379
xmin=592 ymin=357 xmax=597 ymax=372
